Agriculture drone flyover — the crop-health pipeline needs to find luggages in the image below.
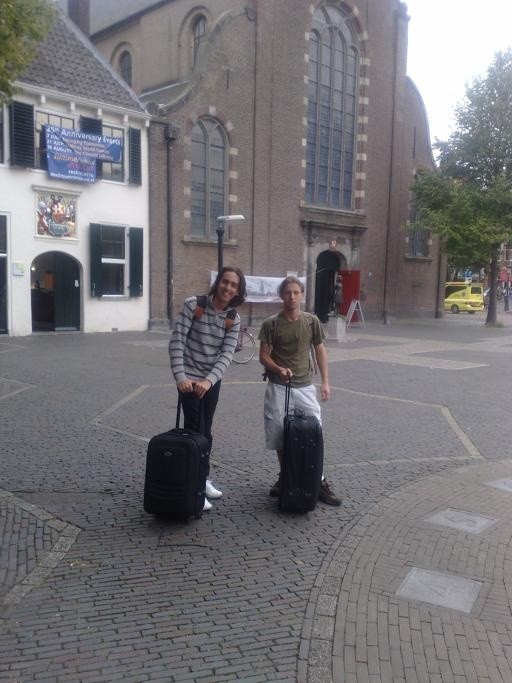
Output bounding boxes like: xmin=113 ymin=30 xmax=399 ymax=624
xmin=280 ymin=372 xmax=324 ymax=513
xmin=142 ymin=385 xmax=210 ymax=521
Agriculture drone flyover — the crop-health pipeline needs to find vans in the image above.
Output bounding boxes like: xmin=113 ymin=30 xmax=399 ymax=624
xmin=444 ymin=281 xmax=485 ymax=313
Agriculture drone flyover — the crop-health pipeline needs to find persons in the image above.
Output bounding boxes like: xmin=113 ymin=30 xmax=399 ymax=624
xmin=332 ymin=275 xmax=343 ymax=315
xmin=257 ymin=276 xmax=341 ymax=506
xmin=168 ymin=266 xmax=247 ymax=510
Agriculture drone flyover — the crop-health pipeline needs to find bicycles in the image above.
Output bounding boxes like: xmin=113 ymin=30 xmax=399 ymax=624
xmin=231 ymin=328 xmax=257 ymax=364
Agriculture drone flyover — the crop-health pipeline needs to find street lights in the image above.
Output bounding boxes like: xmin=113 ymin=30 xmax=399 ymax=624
xmin=215 ymin=214 xmax=246 ymax=274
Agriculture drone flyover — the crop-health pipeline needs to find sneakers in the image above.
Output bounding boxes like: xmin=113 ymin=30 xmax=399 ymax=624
xmin=320 ymin=477 xmax=341 ymax=505
xmin=269 ymin=481 xmax=281 ymax=496
xmin=203 ymin=496 xmax=212 ymax=511
xmin=206 ymin=479 xmax=222 ymax=498
